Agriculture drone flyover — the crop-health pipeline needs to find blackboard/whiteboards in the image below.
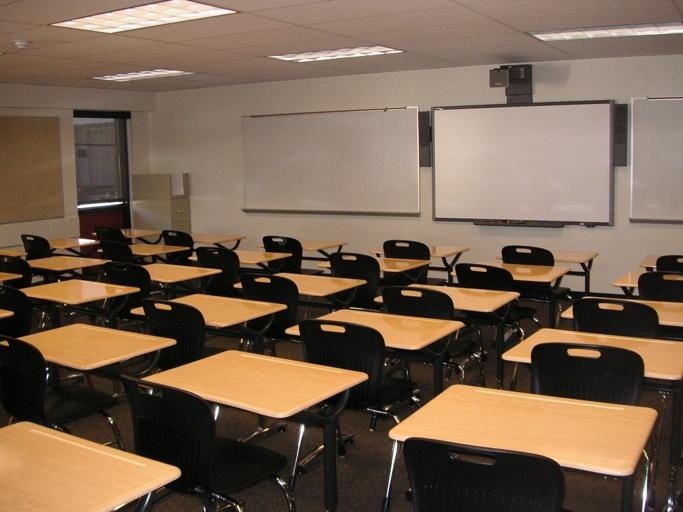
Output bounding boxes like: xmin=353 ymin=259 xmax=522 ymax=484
xmin=432 ymin=99 xmax=615 ymax=227
xmin=240 ymin=106 xmax=420 ymax=216
xmin=629 ymin=97 xmax=683 ymax=223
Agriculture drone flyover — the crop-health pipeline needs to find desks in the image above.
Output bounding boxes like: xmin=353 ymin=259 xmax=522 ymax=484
xmin=0 ymin=421 xmax=182 ymax=512
xmin=384 ymin=383 xmax=658 ymax=512
xmin=138 ymin=350 xmax=369 ymax=507
xmin=500 ymin=326 xmax=683 ymax=508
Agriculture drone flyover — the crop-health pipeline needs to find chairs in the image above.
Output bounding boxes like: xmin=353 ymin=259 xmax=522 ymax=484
xmin=119 ymin=374 xmax=293 ymax=512
xmin=404 ymin=436 xmax=565 ymax=512
xmin=299 ymin=319 xmax=414 ymax=508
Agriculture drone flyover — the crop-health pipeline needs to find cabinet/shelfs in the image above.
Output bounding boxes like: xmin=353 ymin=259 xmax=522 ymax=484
xmin=132 ymin=173 xmax=192 ymax=263
